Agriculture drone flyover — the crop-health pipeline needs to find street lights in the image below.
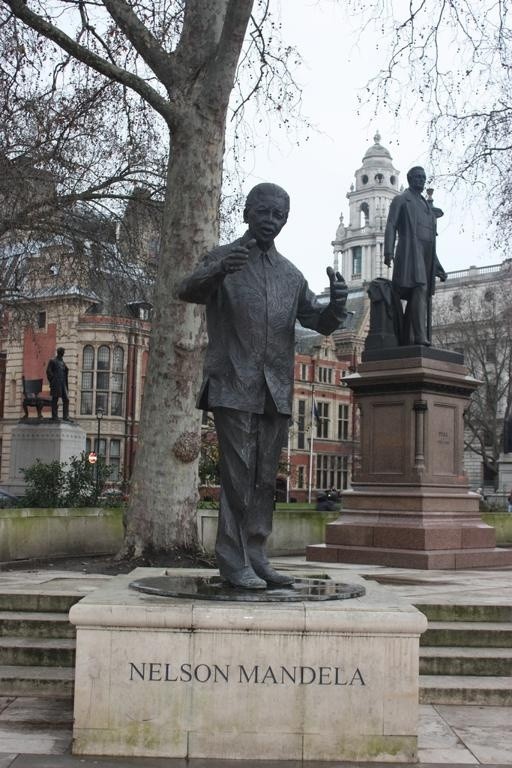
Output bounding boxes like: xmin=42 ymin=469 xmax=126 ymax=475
xmin=95 ymin=406 xmax=104 ymax=489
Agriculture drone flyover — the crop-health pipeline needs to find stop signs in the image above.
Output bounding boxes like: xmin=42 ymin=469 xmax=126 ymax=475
xmin=88 ymin=452 xmax=98 ymax=464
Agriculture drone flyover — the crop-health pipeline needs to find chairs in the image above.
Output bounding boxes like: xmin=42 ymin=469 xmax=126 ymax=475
xmin=22 ymin=375 xmax=59 ymax=418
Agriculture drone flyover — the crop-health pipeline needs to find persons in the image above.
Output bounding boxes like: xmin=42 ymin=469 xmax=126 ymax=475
xmin=384 ymin=166 xmax=448 ymax=346
xmin=46 ymin=347 xmax=74 ymax=422
xmin=178 ymin=182 xmax=350 ymax=589
